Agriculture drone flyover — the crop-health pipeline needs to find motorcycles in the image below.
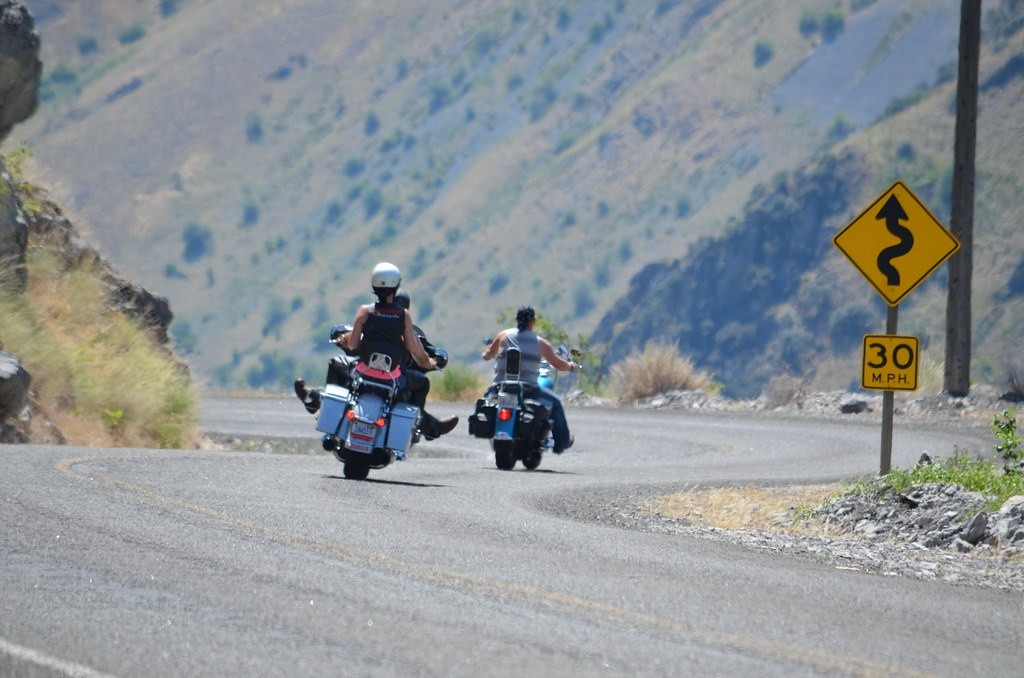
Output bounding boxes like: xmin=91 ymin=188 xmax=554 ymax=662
xmin=316 ymin=323 xmax=447 ymax=479
xmin=467 ymin=336 xmax=583 ymax=471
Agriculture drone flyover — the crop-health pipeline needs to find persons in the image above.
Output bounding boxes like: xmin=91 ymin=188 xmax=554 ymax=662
xmin=294 ymin=262 xmax=459 ymax=441
xmin=483 ymin=306 xmax=577 ymax=453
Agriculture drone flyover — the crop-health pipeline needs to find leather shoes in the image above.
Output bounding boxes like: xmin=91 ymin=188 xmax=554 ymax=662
xmin=552 ymin=432 xmax=575 ymax=454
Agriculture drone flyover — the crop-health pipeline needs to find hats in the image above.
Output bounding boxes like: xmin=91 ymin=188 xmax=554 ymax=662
xmin=515 ymin=305 xmax=536 ymax=322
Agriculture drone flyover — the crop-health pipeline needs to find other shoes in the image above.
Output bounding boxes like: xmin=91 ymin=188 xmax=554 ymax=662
xmin=294 ymin=378 xmax=321 ymax=415
xmin=422 ymin=414 xmax=459 ymax=441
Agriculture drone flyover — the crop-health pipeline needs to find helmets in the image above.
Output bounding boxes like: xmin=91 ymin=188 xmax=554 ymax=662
xmin=371 ymin=262 xmax=401 ymax=288
xmin=395 ymin=289 xmax=410 ymax=310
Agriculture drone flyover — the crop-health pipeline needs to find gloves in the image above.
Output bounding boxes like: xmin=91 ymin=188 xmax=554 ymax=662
xmin=570 ymin=361 xmax=578 ymax=371
